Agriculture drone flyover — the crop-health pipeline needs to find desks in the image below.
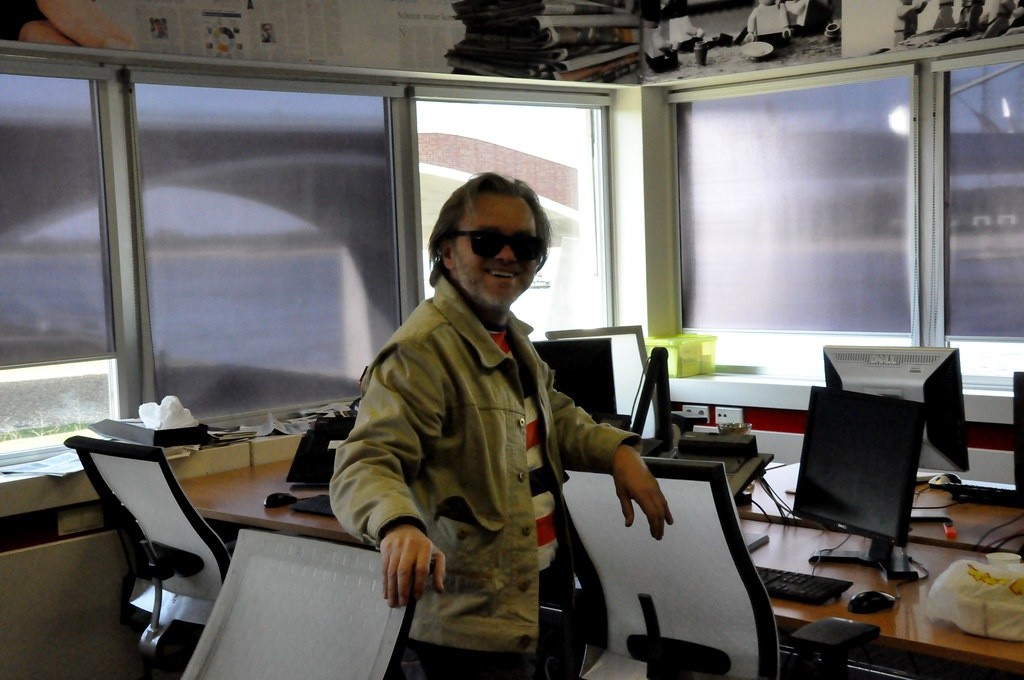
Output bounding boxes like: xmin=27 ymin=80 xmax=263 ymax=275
xmin=177 ymin=460 xmax=1024 ymax=680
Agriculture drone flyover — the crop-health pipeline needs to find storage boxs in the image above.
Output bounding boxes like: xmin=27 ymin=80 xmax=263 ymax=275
xmin=644 ymin=333 xmax=717 ymax=379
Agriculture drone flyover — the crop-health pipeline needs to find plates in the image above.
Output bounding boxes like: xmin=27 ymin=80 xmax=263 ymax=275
xmin=739 ymin=41 xmax=774 ymax=58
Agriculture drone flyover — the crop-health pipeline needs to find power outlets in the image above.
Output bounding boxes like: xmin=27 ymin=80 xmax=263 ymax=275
xmin=681 ymin=404 xmax=710 ymax=424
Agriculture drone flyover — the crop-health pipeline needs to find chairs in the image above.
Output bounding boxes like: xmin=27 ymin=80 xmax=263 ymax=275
xmin=195 ymin=530 xmax=434 ymax=680
xmin=554 ymin=457 xmax=784 ymax=680
xmin=63 ymin=435 xmax=231 ymax=680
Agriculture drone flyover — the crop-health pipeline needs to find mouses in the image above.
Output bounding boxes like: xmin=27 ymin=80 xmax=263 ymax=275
xmin=264 ymin=493 xmax=298 ymax=508
xmin=847 ymin=590 xmax=895 ymax=615
xmin=928 ymin=472 xmax=962 ymax=489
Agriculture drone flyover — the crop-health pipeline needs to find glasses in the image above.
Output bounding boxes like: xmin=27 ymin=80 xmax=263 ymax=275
xmin=453 ymin=229 xmax=544 ymax=264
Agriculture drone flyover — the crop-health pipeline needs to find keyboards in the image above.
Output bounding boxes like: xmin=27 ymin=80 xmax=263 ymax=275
xmin=755 ymin=565 xmax=853 ymax=605
xmin=942 ymin=480 xmax=1023 ymax=509
xmin=292 ymin=494 xmax=336 ymax=516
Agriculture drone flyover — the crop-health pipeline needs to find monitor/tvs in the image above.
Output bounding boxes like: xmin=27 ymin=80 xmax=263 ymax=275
xmin=792 ymin=344 xmax=969 ymax=581
xmin=531 ymin=337 xmax=617 ymax=427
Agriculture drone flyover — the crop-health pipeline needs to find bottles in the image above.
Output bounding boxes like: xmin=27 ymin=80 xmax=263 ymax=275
xmin=693 ymin=41 xmax=708 ymax=66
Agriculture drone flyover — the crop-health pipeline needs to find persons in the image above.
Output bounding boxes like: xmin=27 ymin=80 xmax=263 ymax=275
xmin=330 ymin=172 xmax=675 ymax=680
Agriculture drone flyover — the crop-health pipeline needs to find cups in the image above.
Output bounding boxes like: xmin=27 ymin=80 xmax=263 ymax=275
xmin=1003 ymin=564 xmax=1024 ymax=574
xmin=986 ymin=552 xmax=1022 ymax=566
